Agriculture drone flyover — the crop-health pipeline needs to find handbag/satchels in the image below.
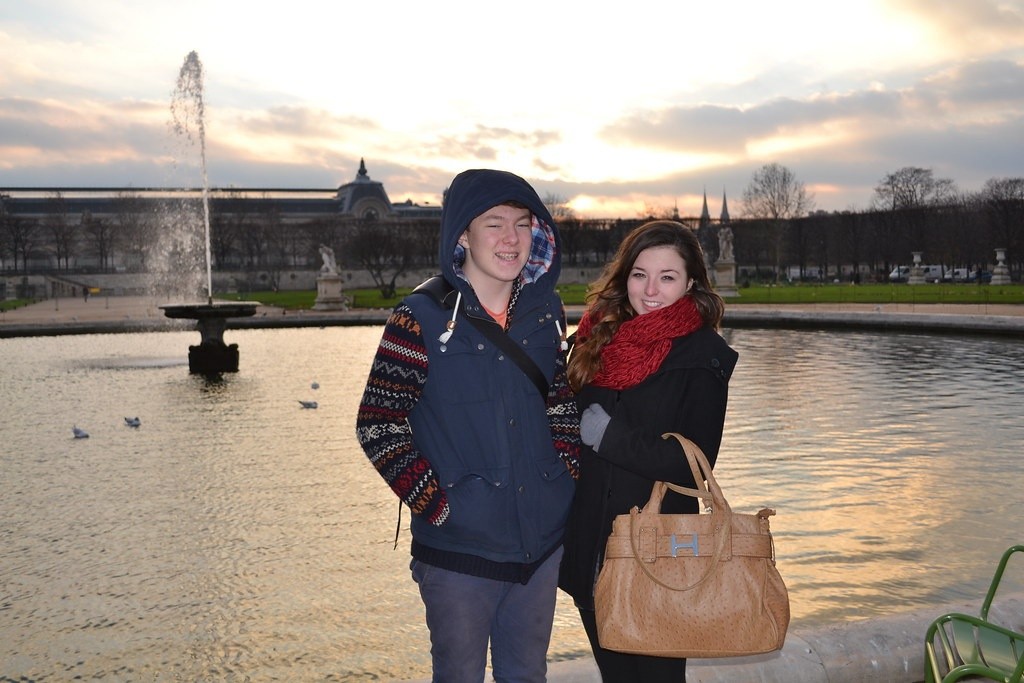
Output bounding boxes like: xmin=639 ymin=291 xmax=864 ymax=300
xmin=594 ymin=431 xmax=789 ymax=657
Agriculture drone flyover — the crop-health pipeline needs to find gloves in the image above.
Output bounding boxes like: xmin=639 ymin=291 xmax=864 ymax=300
xmin=579 ymin=403 xmax=611 ymax=448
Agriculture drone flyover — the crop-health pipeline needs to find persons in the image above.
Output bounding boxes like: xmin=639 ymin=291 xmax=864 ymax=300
xmin=319 ymin=243 xmax=336 ymax=272
xmin=556 ymin=220 xmax=739 ymax=683
xmin=717 ymin=224 xmax=734 ymax=260
xmin=356 ymin=170 xmax=582 ymax=683
xmin=83 ymin=286 xmax=88 ymax=302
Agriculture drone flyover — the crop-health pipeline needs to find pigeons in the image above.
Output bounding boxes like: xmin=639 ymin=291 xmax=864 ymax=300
xmin=299 ymin=401 xmax=318 ymax=409
xmin=72 ymin=425 xmax=89 ymax=438
xmin=125 ymin=417 xmax=141 ymax=427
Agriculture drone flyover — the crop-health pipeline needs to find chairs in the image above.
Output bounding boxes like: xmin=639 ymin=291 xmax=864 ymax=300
xmin=922 ymin=546 xmax=1024 ymax=683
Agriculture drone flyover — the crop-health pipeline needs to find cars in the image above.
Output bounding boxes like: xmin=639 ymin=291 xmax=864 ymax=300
xmin=888 ymin=262 xmax=993 ymax=284
